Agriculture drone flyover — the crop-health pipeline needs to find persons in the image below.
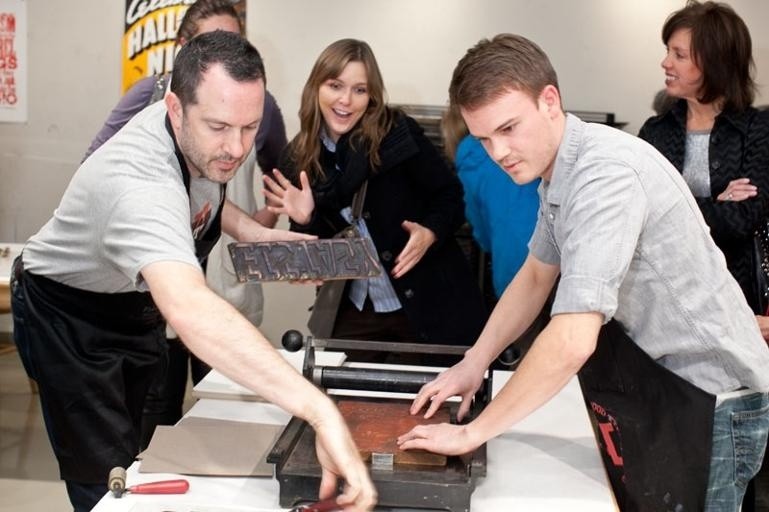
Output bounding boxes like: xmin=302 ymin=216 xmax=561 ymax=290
xmin=9 ymin=27 xmax=381 ymax=512
xmin=753 ymin=313 xmax=769 ymax=345
xmin=441 ymin=103 xmax=544 ymax=300
xmin=635 ymin=0 xmax=768 ymax=316
xmin=397 ymin=33 xmax=768 ymax=512
xmin=81 ymin=0 xmax=290 ymax=455
xmin=257 ymin=37 xmax=472 ymax=366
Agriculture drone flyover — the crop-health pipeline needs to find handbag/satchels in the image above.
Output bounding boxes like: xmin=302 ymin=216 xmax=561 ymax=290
xmin=746 ymin=229 xmax=769 ymax=314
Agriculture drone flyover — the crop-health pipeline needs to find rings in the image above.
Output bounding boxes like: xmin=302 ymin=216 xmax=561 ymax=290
xmin=728 ymin=191 xmax=733 ymax=200
